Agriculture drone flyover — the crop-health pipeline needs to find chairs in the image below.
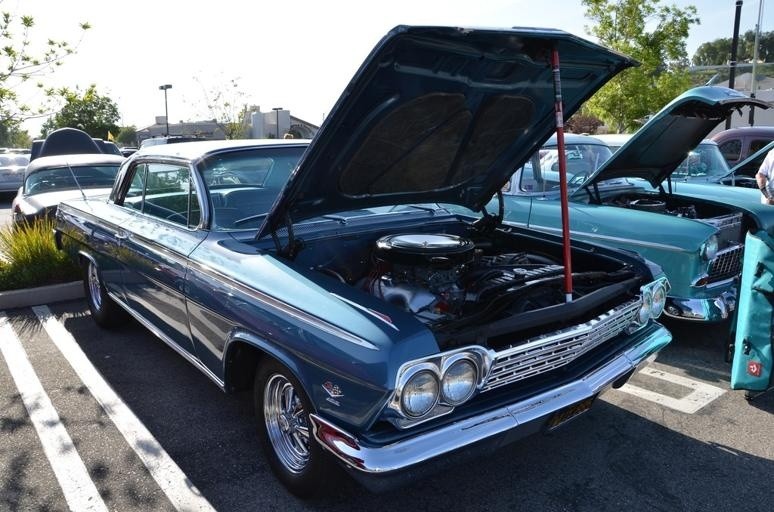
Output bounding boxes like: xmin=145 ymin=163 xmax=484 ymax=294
xmin=192 ymin=207 xmax=248 ymax=232
xmin=520 ymin=179 xmax=539 ymax=193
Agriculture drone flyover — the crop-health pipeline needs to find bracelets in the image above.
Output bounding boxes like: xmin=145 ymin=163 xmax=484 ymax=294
xmin=760 ymin=186 xmax=770 ymax=199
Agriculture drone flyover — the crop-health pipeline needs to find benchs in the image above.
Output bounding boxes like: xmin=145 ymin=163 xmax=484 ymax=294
xmin=135 ymin=188 xmax=280 ymax=218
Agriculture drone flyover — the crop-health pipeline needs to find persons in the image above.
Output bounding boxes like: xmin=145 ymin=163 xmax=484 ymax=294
xmin=754 ymin=148 xmax=774 ymax=207
